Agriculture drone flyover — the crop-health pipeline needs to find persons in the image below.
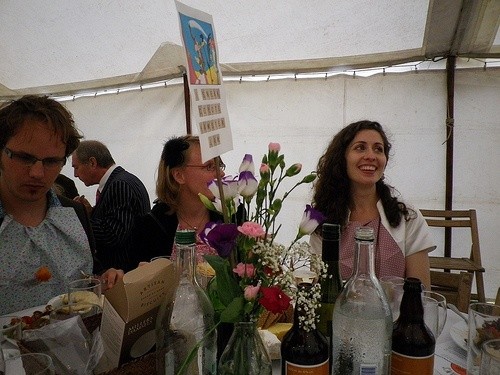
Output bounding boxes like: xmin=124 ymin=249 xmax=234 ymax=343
xmin=73 ymin=142 xmax=150 ymax=270
xmin=0 ymin=95 xmax=125 ymax=316
xmin=54 ymin=174 xmax=79 ymax=200
xmin=127 ymin=136 xmax=226 ymax=263
xmin=310 ymin=120 xmax=437 ymax=297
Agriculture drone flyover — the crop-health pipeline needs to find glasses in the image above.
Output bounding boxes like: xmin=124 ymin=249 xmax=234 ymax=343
xmin=2 ymin=145 xmax=66 ymax=171
xmin=182 ymin=162 xmax=226 ymax=172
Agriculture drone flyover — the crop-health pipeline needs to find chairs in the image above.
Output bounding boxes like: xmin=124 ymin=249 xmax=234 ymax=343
xmin=417 ymin=208 xmax=486 ymax=315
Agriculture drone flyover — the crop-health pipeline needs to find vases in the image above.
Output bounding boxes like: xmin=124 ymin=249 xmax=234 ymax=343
xmin=216 ymin=321 xmax=273 ymax=375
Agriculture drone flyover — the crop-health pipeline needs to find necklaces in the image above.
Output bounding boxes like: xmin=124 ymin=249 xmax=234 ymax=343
xmin=180 ymin=210 xmax=208 ymax=231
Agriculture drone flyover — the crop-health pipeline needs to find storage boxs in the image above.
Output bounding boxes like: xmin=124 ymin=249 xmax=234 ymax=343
xmin=101 ymin=257 xmax=173 ymax=368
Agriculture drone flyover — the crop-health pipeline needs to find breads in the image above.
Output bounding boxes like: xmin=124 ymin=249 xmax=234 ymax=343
xmin=17 ymin=303 xmax=104 ymax=352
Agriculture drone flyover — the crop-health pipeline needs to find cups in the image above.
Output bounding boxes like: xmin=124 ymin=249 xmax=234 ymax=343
xmin=466 ymin=303 xmax=500 ymax=375
xmin=0 ymin=352 xmax=55 ymax=375
xmin=0 ymin=315 xmax=22 ymax=361
xmin=68 ymin=278 xmax=102 ymax=333
xmin=479 ymin=340 xmax=500 ymax=375
xmin=151 ymin=256 xmax=176 ymax=266
xmin=379 ymin=276 xmax=447 ymax=340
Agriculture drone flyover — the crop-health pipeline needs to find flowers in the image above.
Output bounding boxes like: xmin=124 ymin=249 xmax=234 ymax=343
xmin=176 ymin=143 xmax=326 ymax=375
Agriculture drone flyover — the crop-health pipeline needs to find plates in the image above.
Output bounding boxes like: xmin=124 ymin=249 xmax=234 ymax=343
xmin=47 ymin=291 xmax=96 ymax=314
xmin=450 ymin=317 xmax=496 ymax=352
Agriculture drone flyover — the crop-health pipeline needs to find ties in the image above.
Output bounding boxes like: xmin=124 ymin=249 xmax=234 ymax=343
xmin=95 ymin=188 xmax=101 ymax=205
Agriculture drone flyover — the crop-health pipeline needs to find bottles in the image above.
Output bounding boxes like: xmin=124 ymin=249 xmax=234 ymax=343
xmin=313 ymin=223 xmax=343 ymax=340
xmin=331 ymin=227 xmax=393 ymax=375
xmin=280 ymin=282 xmax=330 ymax=375
xmin=389 ymin=277 xmax=435 ymax=375
xmin=155 ymin=229 xmax=216 ymax=375
